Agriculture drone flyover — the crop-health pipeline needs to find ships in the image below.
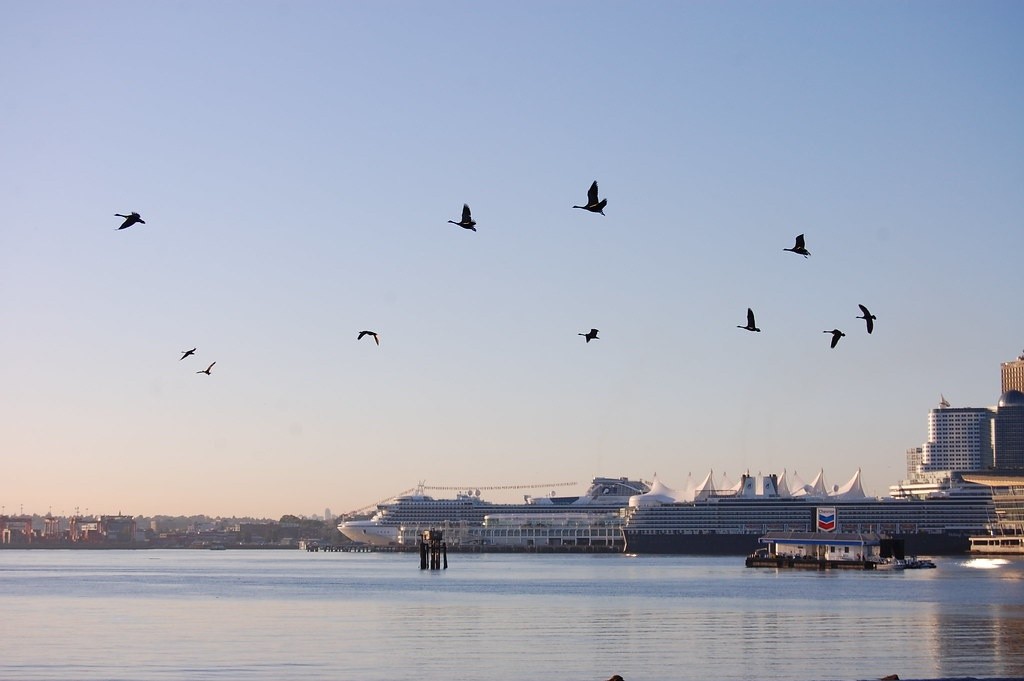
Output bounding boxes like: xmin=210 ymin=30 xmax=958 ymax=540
xmin=337 ymin=475 xmax=652 ymax=553
xmin=618 ymin=470 xmax=1002 ymax=559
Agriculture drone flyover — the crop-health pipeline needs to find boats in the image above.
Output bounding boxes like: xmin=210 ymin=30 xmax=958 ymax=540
xmin=744 ymin=530 xmax=938 ymax=569
xmin=958 ymin=473 xmax=1024 ymax=557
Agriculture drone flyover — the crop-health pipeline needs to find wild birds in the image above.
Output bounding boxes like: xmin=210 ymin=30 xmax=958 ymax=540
xmin=577 ymin=329 xmax=600 ymax=343
xmin=572 ymin=180 xmax=608 ymax=216
xmin=196 ymin=362 xmax=216 ymax=375
xmin=357 ymin=330 xmax=379 ymax=346
xmin=737 ymin=308 xmax=761 ymax=332
xmin=114 ymin=212 xmax=146 ymax=230
xmin=447 ymin=204 xmax=476 ymax=232
xmin=856 ymin=304 xmax=876 ymax=334
xmin=822 ymin=329 xmax=845 ymax=348
xmin=783 ymin=234 xmax=811 ymax=258
xmin=180 ymin=348 xmax=196 ymax=360
